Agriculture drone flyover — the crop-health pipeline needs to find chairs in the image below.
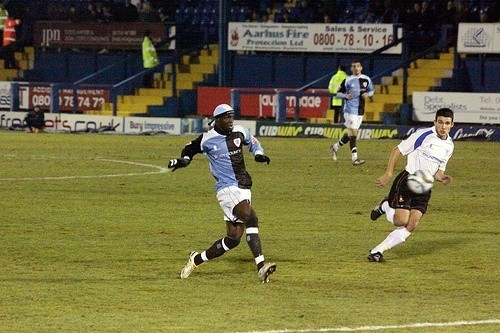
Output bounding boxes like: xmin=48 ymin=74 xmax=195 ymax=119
xmin=174 ymin=0 xmax=250 ymax=27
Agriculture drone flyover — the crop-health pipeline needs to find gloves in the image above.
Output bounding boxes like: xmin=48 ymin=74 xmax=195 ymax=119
xmin=255 ymin=153 xmax=270 ymax=164
xmin=168 ymin=157 xmax=190 ymax=172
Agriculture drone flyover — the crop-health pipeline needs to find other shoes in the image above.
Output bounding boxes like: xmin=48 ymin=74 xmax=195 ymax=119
xmin=367 ymin=249 xmax=387 ymax=262
xmin=330 ymin=144 xmax=337 ymax=160
xmin=353 ymin=158 xmax=366 ymax=165
xmin=371 ymin=196 xmax=389 ymax=220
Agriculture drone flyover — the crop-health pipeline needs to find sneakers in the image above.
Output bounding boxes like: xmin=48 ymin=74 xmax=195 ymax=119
xmin=258 ymin=262 xmax=277 ymax=283
xmin=181 ymin=250 xmax=199 ymax=278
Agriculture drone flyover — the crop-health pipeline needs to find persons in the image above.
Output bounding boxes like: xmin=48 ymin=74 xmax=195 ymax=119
xmin=366 ymin=108 xmax=455 ymax=263
xmin=0 ymin=0 xmax=500 ymax=134
xmin=167 ymin=104 xmax=277 ymax=284
xmin=331 ymin=60 xmax=375 ymax=166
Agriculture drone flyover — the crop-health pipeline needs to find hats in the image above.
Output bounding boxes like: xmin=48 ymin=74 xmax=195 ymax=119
xmin=213 ymin=103 xmax=235 ymax=118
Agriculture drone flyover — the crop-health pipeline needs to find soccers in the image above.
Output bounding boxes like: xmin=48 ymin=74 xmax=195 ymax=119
xmin=407 ymin=168 xmax=435 ymax=194
